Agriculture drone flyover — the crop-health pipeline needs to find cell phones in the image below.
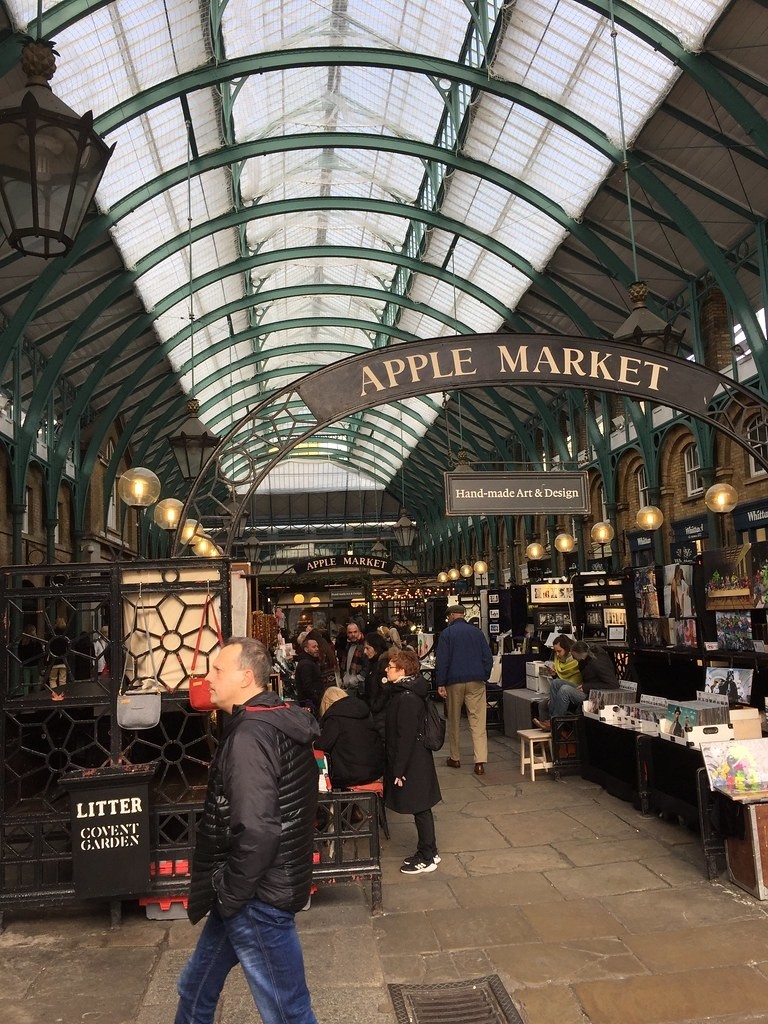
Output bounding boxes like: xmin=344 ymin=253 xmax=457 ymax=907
xmin=544 ymin=664 xmax=558 ymax=674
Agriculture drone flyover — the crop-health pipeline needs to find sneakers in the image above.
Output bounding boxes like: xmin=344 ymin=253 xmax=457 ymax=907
xmin=400 ymin=857 xmax=438 ymax=874
xmin=404 ymin=851 xmax=441 ymax=864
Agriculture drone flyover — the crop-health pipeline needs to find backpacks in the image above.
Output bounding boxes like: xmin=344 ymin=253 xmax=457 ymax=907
xmin=417 ymin=695 xmax=446 ymax=751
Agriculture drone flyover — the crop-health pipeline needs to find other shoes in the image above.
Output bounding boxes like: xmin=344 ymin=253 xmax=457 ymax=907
xmin=350 ymin=810 xmax=363 ymax=823
xmin=568 ymin=744 xmax=576 ymax=757
xmin=561 ymin=730 xmax=575 ymax=741
xmin=532 ymin=718 xmax=552 ymax=731
xmin=559 ymin=745 xmax=567 ymax=758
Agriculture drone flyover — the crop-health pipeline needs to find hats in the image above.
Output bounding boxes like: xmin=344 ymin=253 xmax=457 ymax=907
xmin=446 ymin=605 xmax=464 ymax=616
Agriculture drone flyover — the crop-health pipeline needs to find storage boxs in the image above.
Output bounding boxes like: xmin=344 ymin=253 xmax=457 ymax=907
xmin=582 ymin=698 xmax=763 ymax=752
xmin=525 ymin=660 xmax=552 ymax=692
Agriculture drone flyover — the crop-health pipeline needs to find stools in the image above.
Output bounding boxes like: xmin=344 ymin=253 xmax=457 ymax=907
xmin=342 ymin=777 xmax=391 ymax=846
xmin=517 ymin=726 xmax=555 ymax=782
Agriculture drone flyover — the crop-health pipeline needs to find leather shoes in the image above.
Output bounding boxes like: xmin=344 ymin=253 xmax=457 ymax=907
xmin=474 ymin=764 xmax=485 ymax=775
xmin=447 ymin=757 xmax=460 ymax=768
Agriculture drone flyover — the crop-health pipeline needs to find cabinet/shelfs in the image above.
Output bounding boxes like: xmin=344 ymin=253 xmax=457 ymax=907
xmin=581 ymin=648 xmax=768 ymax=879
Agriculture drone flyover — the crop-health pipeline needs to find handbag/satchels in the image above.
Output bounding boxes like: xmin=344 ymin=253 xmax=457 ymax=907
xmin=117 ymin=694 xmax=161 ymax=730
xmin=189 ymin=677 xmax=221 ymax=712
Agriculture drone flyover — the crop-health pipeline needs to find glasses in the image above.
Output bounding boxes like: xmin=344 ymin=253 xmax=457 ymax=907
xmin=389 ymin=665 xmax=397 ymax=669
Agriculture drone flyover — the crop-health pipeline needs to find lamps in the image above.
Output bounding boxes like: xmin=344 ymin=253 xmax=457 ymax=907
xmin=705 ymin=483 xmax=738 ymax=545
xmin=554 ymin=535 xmax=575 ymax=576
xmin=636 ymin=505 xmax=664 ymax=567
xmin=370 ymin=250 xmax=473 ymax=558
xmin=0 ymin=0 xmax=118 ymax=263
xmin=118 ymin=123 xmax=264 ymax=568
xmin=607 ymin=0 xmax=686 ymax=357
xmin=526 ymin=543 xmax=545 ymax=580
xmin=437 ymin=561 xmax=488 ymax=595
xmin=591 ymin=522 xmax=614 ymax=569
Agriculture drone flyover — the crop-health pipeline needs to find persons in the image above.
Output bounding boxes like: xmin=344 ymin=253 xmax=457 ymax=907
xmin=668 ymin=706 xmax=682 ymax=736
xmin=174 ymin=637 xmax=321 ymax=1024
xmin=669 ymin=565 xmax=691 ymax=618
xmin=285 ymin=618 xmax=418 ymax=821
xmin=532 ymin=635 xmax=619 ymax=760
xmin=381 ymin=650 xmax=442 ymax=875
xmin=435 ymin=606 xmax=494 ymax=775
xmin=17 ymin=617 xmax=108 ymax=696
